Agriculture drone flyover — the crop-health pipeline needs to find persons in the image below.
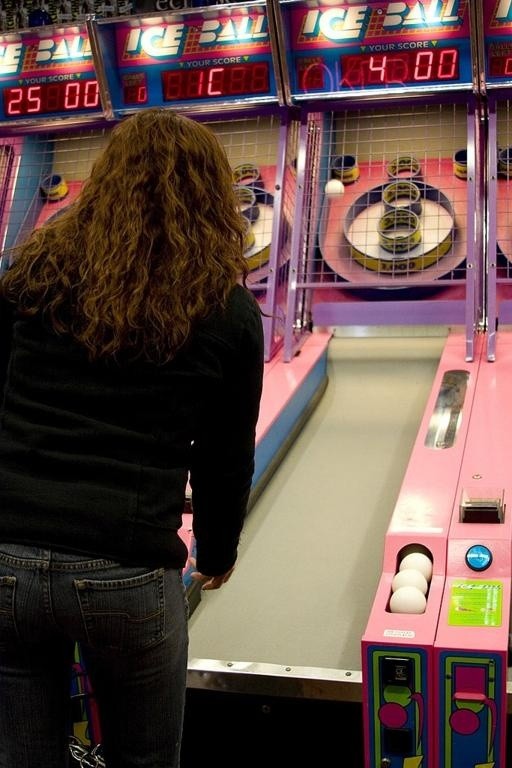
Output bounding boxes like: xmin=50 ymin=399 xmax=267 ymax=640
xmin=0 ymin=107 xmax=276 ymax=767
